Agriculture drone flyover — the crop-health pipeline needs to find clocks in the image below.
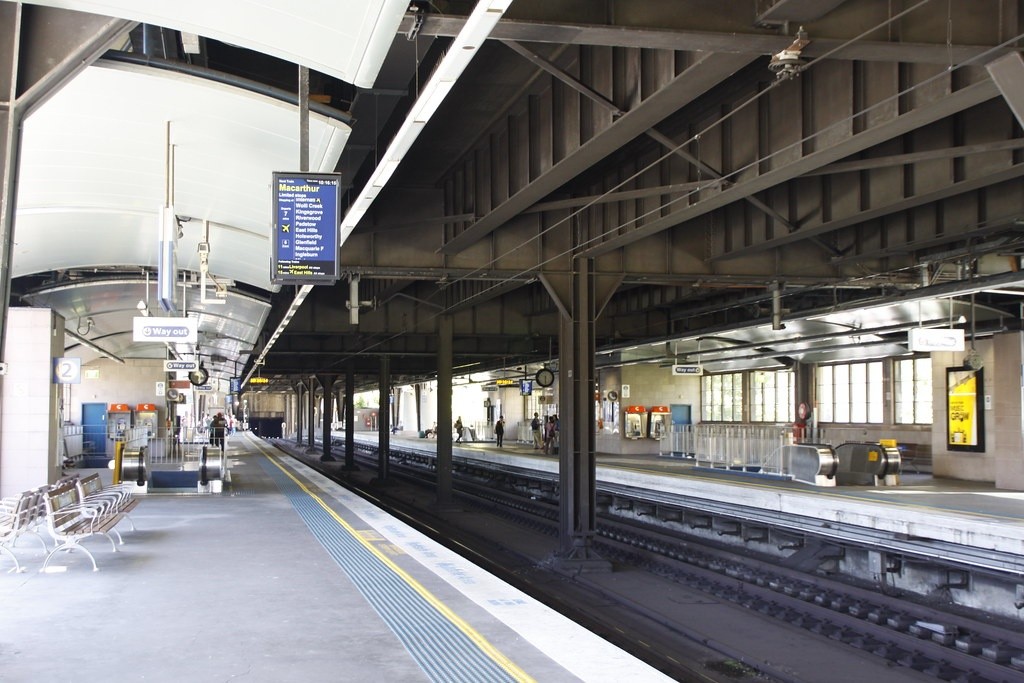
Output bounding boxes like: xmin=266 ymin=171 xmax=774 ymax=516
xmin=535 ymin=369 xmax=555 ymax=388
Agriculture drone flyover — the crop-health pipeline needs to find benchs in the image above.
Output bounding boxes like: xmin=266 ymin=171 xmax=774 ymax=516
xmin=0 ymin=473 xmax=142 ymax=574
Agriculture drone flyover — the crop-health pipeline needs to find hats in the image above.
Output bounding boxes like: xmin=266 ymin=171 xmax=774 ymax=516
xmin=218 ymin=413 xmax=222 ymax=417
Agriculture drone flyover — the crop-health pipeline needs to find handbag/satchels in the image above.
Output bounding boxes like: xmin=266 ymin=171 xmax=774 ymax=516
xmin=454 ymin=423 xmax=462 ymax=429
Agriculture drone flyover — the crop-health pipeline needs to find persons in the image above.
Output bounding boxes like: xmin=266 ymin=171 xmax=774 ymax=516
xmin=531 ymin=411 xmax=544 ymax=449
xmin=455 ymin=416 xmax=463 ymax=444
xmin=544 ymin=414 xmax=560 ymax=455
xmin=432 ymin=422 xmax=436 ymax=438
xmin=494 ymin=416 xmax=505 ymax=447
xmin=205 ymin=412 xmax=237 ymax=452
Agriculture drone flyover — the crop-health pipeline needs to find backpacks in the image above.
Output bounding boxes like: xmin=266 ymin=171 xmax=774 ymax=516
xmin=549 ymin=423 xmax=555 ymax=436
